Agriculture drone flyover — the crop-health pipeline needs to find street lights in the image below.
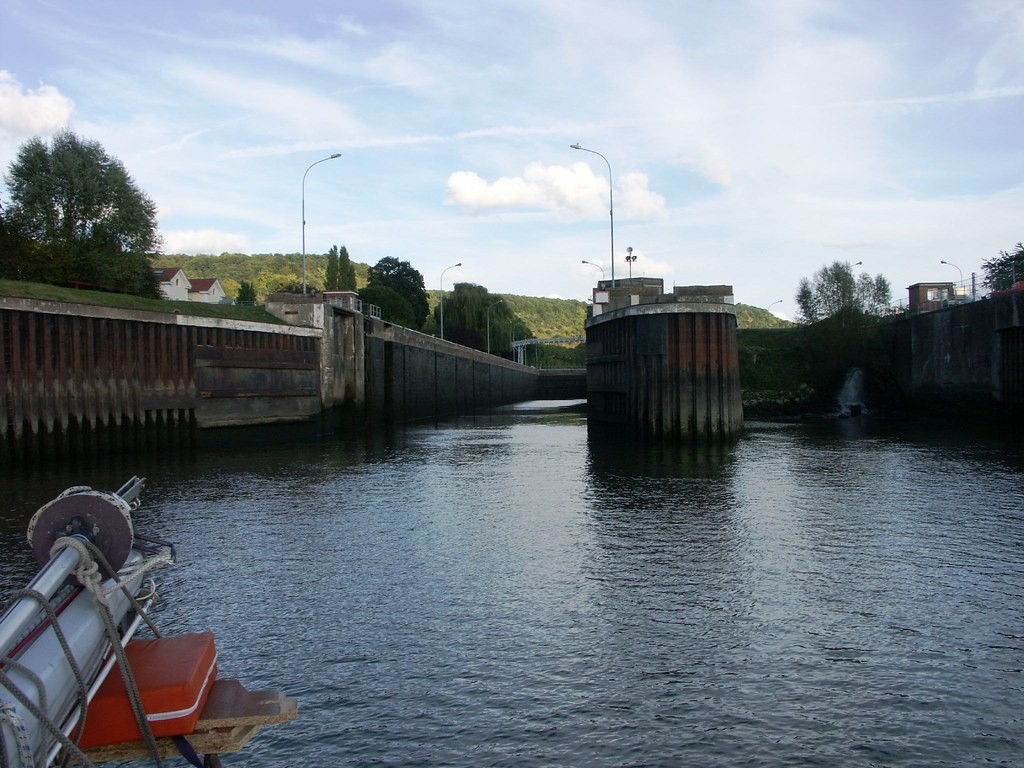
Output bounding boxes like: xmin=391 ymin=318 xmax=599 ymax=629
xmin=440 ymin=263 xmax=461 ymax=340
xmin=582 ymin=261 xmax=604 ymax=279
xmin=768 ymin=299 xmax=783 ymax=327
xmin=570 ymin=142 xmax=615 ymax=289
xmin=301 ymin=153 xmax=342 ymax=294
xmin=487 ymin=300 xmax=503 ymax=353
xmin=941 ymin=261 xmax=963 ymax=288
